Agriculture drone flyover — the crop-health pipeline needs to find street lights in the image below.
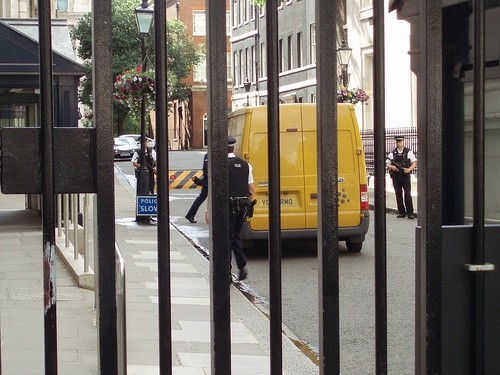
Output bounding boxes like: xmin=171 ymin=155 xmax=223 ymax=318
xmin=336 ymin=38 xmax=353 ymax=92
xmin=132 ymin=0 xmax=156 ymax=224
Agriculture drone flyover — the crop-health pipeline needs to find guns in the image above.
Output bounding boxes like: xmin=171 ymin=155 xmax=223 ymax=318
xmin=386 ymin=155 xmax=406 ymax=171
xmin=238 ymin=196 xmax=257 ymax=217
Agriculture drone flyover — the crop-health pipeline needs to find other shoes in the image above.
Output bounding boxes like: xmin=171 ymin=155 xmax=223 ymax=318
xmin=408 ymin=214 xmax=415 ymax=218
xmin=397 ymin=214 xmax=406 ymax=218
xmin=239 ymin=267 xmax=248 ymax=281
xmin=185 ymin=216 xmax=196 ymax=223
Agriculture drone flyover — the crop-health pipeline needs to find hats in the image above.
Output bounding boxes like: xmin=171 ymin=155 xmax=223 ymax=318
xmin=138 ymin=134 xmax=149 ymax=141
xmin=394 ymin=135 xmax=404 ymax=141
xmin=228 ymin=136 xmax=237 ymax=147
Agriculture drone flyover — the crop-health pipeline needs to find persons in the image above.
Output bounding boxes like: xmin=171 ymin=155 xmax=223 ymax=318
xmin=131 ymin=136 xmax=157 ymax=193
xmin=385 ymin=136 xmax=417 ymax=219
xmin=185 ymin=152 xmax=208 ymax=223
xmin=227 ymin=137 xmax=255 ymax=281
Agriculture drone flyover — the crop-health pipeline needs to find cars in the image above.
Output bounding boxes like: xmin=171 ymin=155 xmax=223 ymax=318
xmin=113 ymin=137 xmax=139 ymax=159
xmin=119 ymin=134 xmax=157 ymax=150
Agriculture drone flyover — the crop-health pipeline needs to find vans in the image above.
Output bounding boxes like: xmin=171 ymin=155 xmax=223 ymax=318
xmin=227 ymin=102 xmax=370 ymax=253
